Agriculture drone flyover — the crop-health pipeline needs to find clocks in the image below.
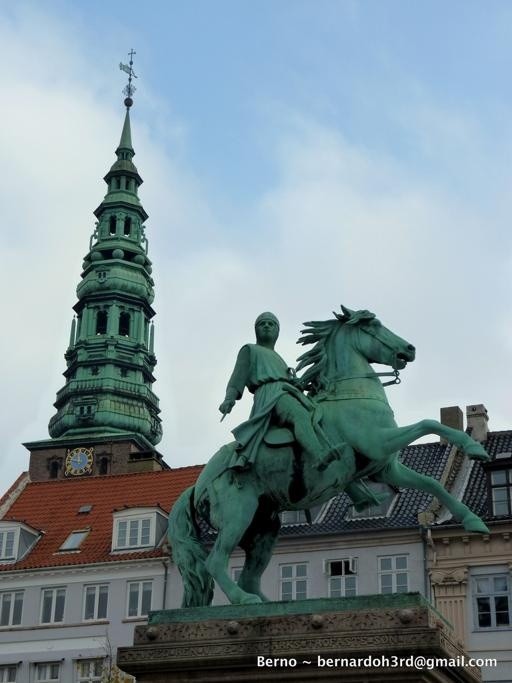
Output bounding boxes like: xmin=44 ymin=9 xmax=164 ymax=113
xmin=64 ymin=447 xmax=93 ymax=476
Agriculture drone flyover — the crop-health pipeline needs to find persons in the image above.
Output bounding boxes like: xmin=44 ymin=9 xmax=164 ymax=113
xmin=215 ymin=310 xmax=392 ymax=514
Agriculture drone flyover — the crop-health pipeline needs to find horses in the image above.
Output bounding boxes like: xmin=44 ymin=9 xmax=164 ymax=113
xmin=166 ymin=304 xmax=493 ymax=605
